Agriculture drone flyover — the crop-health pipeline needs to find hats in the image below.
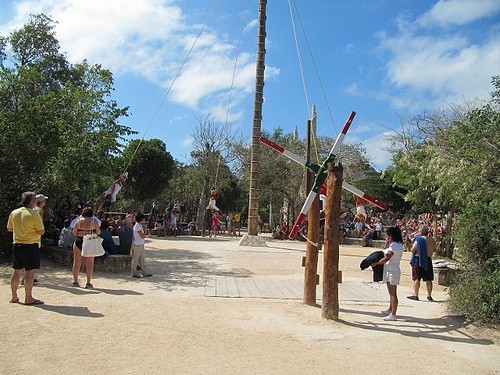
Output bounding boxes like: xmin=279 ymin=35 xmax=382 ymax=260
xmin=36 ymin=193 xmax=49 ymax=201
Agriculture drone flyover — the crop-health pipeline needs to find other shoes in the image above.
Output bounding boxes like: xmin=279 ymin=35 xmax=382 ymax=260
xmin=20 ymin=277 xmax=39 ymax=284
xmin=382 ymin=313 xmax=396 ymax=320
xmin=132 ymin=273 xmax=141 ymax=277
xmin=407 ymin=295 xmax=418 ymax=300
xmin=143 ymin=273 xmax=152 ymax=276
xmin=427 ymin=296 xmax=433 ymax=301
xmin=85 ymin=283 xmax=93 ymax=288
xmin=381 ymin=310 xmax=391 ymax=314
xmin=72 ymin=281 xmax=80 ymax=286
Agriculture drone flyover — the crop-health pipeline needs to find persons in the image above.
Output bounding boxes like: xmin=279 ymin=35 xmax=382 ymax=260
xmin=59 ymin=199 xmax=137 ymax=255
xmin=147 ymin=201 xmax=183 ymax=235
xmin=207 ymin=211 xmax=241 ymax=238
xmin=319 ymin=162 xmax=334 ymax=214
xmin=131 ymin=214 xmax=153 ymax=278
xmin=340 ymin=191 xmax=461 ymax=250
xmin=74 ymin=207 xmax=102 ymax=289
xmin=205 ymin=188 xmax=223 ymax=215
xmin=6 ymin=191 xmax=49 ymax=304
xmin=188 ymin=218 xmax=198 ymax=236
xmin=371 ymin=226 xmax=403 ymax=321
xmin=273 ymin=220 xmax=310 ymax=241
xmin=406 ymin=226 xmax=437 ymax=301
xmin=97 ymin=172 xmax=129 ymax=216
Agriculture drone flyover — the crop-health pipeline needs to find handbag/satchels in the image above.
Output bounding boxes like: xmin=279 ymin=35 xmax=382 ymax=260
xmin=103 ymin=242 xmax=130 ymax=255
xmin=81 ymin=233 xmax=106 ymax=257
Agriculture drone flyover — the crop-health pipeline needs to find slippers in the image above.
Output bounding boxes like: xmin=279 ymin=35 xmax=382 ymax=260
xmin=25 ymin=299 xmax=45 ymax=304
xmin=9 ymin=297 xmax=19 ymax=302
